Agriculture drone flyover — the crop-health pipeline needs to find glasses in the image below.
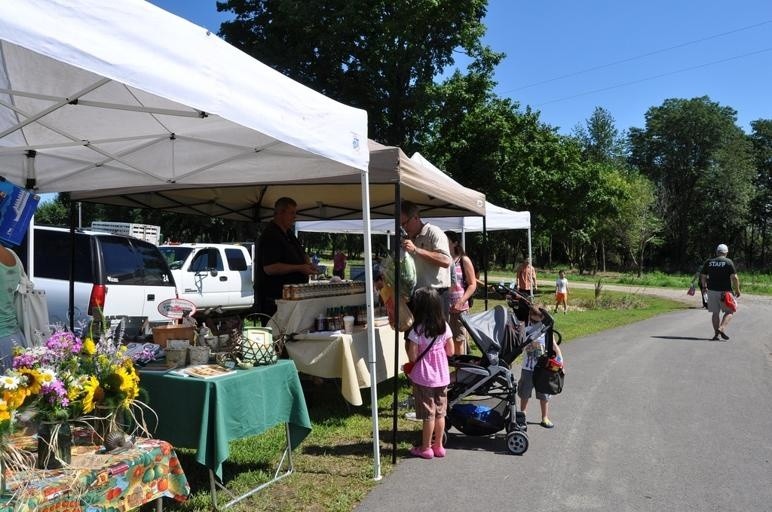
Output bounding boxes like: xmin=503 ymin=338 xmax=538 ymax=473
xmin=400 ymin=215 xmax=413 ymax=228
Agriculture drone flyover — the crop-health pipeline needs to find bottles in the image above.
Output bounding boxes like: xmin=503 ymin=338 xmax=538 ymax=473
xmin=499 ymin=279 xmax=504 ymax=289
xmin=283 ymin=279 xmax=365 ymax=300
xmin=703 ymin=290 xmax=708 ymax=303
xmin=327 ymin=302 xmax=387 ymax=330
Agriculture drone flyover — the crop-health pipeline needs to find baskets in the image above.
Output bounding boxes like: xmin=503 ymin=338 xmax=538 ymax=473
xmin=229 ymin=313 xmax=285 ymax=365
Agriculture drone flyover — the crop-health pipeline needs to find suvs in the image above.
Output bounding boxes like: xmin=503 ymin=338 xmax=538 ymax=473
xmin=9 ymin=226 xmax=181 ymax=339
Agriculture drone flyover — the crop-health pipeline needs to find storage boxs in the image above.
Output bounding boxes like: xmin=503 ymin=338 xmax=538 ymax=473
xmin=152 ymin=324 xmax=195 ymax=349
xmin=0 ymin=176 xmax=40 ymax=248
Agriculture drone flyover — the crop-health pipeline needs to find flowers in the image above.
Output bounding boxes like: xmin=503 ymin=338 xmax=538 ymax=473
xmin=0 ymin=321 xmax=146 ymax=421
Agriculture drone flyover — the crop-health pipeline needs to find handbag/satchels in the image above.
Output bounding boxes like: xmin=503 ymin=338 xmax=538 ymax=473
xmin=532 ymin=352 xmax=564 ymax=395
xmin=462 ymin=276 xmax=478 ymax=297
xmin=404 ymin=361 xmax=415 ymax=374
xmin=5 ymin=245 xmax=49 ymax=348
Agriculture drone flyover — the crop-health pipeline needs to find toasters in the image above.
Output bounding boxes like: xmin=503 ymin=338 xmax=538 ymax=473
xmin=510 ymin=278 xmax=515 ymax=288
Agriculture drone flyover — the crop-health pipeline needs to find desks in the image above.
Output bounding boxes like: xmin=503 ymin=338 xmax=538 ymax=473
xmin=316 ymin=265 xmax=327 ymax=276
xmin=0 ymin=422 xmax=190 ymax=512
xmin=138 ymin=359 xmax=312 ymax=511
xmin=266 ymin=292 xmax=415 ymax=416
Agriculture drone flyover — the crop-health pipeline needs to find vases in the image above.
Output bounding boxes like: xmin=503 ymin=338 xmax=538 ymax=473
xmin=95 ymin=405 xmax=114 ymax=446
xmin=38 ymin=419 xmax=71 ymax=469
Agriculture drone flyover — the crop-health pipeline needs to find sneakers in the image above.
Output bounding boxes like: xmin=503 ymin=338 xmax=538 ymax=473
xmin=431 ymin=444 xmax=446 ymax=457
xmin=391 ymin=398 xmax=415 ymax=409
xmin=410 ymin=444 xmax=434 ymax=458
xmin=541 ymin=421 xmax=554 ymax=428
xmin=716 ymin=328 xmax=729 ymax=339
xmin=404 ymin=412 xmax=424 ymax=422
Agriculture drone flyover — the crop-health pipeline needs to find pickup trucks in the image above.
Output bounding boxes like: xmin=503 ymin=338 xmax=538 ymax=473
xmin=157 ymin=239 xmax=256 ymax=313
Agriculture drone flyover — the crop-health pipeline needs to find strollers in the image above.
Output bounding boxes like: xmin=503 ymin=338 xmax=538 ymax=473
xmin=412 ymin=309 xmax=554 ymax=457
xmin=490 ymin=284 xmax=562 ymax=346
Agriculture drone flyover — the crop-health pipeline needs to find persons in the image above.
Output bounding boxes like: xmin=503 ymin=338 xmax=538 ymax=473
xmin=311 ymin=251 xmax=333 ymax=278
xmin=1 ymin=244 xmax=29 ymax=378
xmin=407 ymin=286 xmax=454 ymax=459
xmin=402 ymin=201 xmax=451 ymax=361
xmin=333 ymin=246 xmax=347 ymax=280
xmin=251 ymin=198 xmax=319 ymax=326
xmin=553 ymin=270 xmax=571 ymax=314
xmin=690 ymin=243 xmax=740 ymax=341
xmin=517 ymin=302 xmax=564 ymax=429
xmin=444 ymin=230 xmax=477 ymax=357
xmin=515 ymin=258 xmax=537 ymax=303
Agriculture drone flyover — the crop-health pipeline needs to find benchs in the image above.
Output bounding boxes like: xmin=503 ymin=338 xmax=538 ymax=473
xmin=478 ymin=282 xmax=510 ymax=299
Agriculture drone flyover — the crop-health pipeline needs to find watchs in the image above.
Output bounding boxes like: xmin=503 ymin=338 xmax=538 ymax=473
xmin=412 ymin=247 xmax=419 ymax=254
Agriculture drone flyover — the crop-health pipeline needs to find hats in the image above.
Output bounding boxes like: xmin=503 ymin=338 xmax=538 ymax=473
xmin=717 ymin=244 xmax=729 ymax=254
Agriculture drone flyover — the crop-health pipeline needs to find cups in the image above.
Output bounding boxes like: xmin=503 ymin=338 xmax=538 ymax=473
xmin=343 ymin=315 xmax=356 ymax=335
